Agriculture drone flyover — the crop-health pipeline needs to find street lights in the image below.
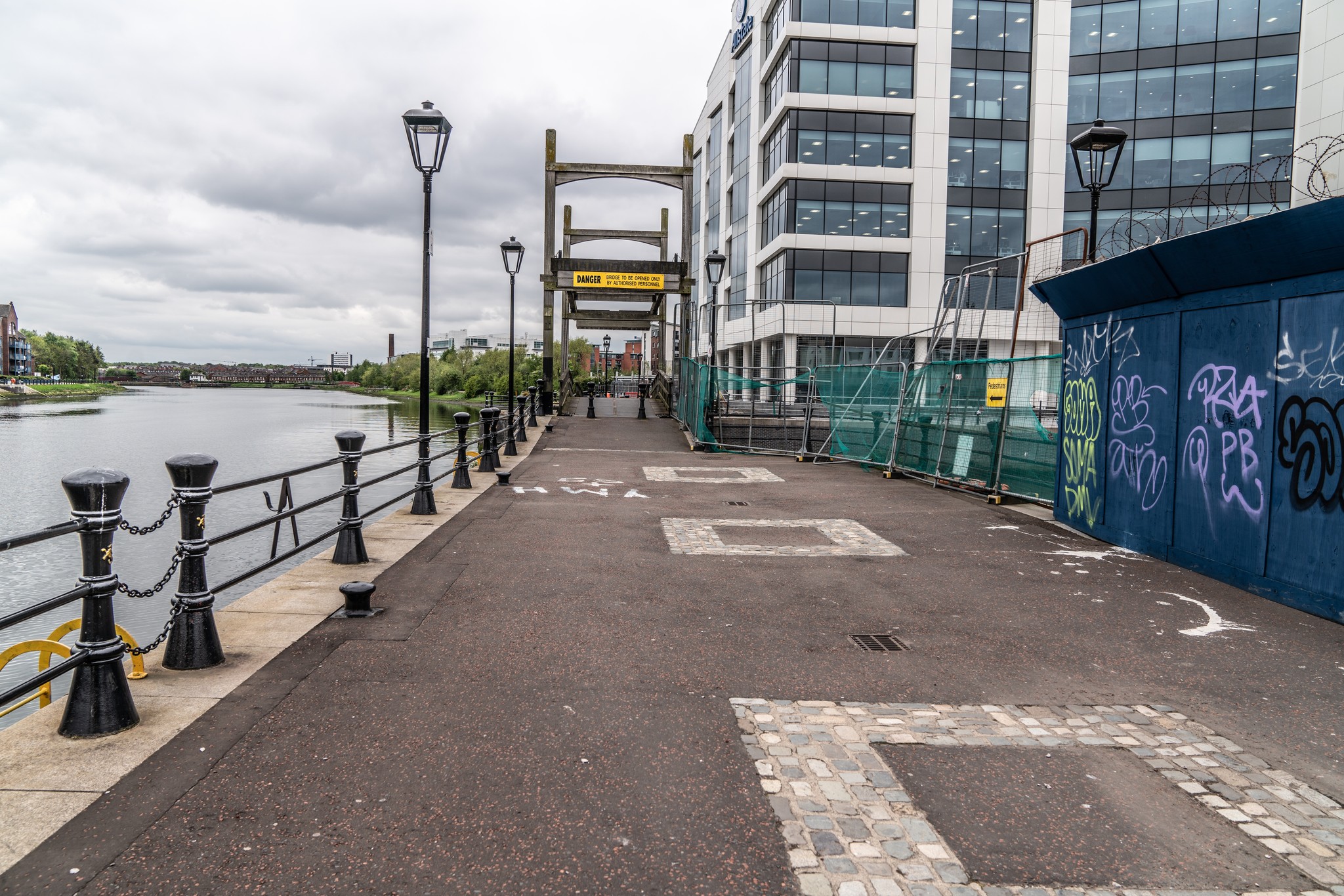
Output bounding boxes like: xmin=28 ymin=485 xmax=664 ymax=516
xmin=353 ymin=372 xmax=368 ymax=388
xmin=346 ymin=374 xmax=346 ymax=381
xmin=400 ymin=99 xmax=454 ymax=517
xmin=17 ymin=361 xmax=21 ymax=380
xmin=637 ymin=352 xmax=643 ymax=398
xmin=35 ymin=362 xmax=39 ymax=385
xmin=94 ymin=363 xmax=101 ymax=384
xmin=466 ymin=374 xmax=468 ymax=382
xmin=539 ymin=371 xmax=542 ymax=379
xmin=65 ymin=364 xmax=68 ymax=384
xmin=47 ymin=359 xmax=50 ymax=375
xmin=613 ymin=366 xmax=617 ymax=398
xmin=602 ymin=334 xmax=611 ymax=397
xmin=704 ymin=249 xmax=727 ymax=453
xmin=526 ymin=372 xmax=529 ymax=392
xmin=1070 ymin=117 xmax=1129 ymax=264
xmin=147 ymin=372 xmax=261 ymax=384
xmin=75 ymin=365 xmax=78 ymax=380
xmin=81 ymin=366 xmax=86 ymax=380
xmin=274 ymin=373 xmax=334 ymax=386
xmin=501 ymin=237 xmax=526 ymax=456
xmin=598 ymin=369 xmax=602 ymax=393
xmin=51 ymin=363 xmax=55 ymax=384
xmin=498 ymin=373 xmax=501 ymax=381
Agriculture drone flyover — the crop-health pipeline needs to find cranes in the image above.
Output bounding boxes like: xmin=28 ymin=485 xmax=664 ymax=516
xmin=212 ymin=360 xmax=238 ymax=367
xmin=294 ymin=356 xmax=323 ymax=366
